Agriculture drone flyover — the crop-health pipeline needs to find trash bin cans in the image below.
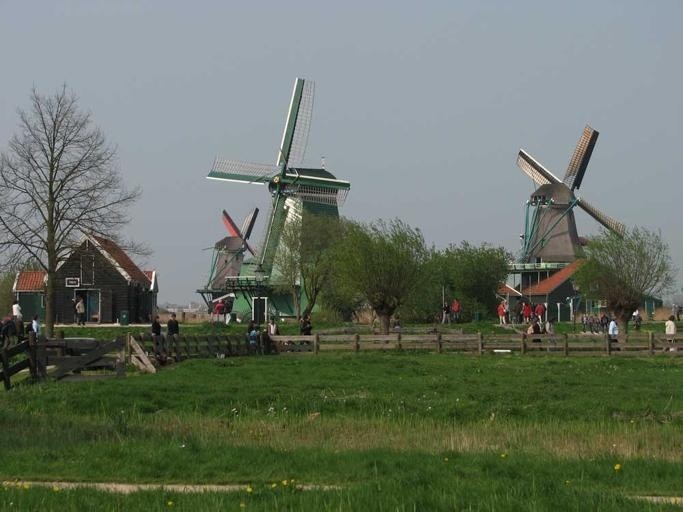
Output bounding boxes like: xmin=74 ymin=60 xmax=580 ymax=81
xmin=120 ymin=310 xmax=129 ymax=325
xmin=228 ymin=313 xmax=236 ymax=325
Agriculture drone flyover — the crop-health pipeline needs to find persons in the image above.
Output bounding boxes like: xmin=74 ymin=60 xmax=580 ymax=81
xmin=633 ymin=313 xmax=642 ymax=329
xmin=299 ymin=312 xmax=312 ymax=345
xmin=268 ymin=316 xmax=279 ymax=335
xmin=672 ymin=304 xmax=680 ymax=321
xmin=662 ymin=315 xmax=677 ymax=352
xmin=12 ymin=301 xmax=23 ymax=320
xmin=75 ymin=298 xmax=85 ymax=326
xmin=167 ymin=314 xmax=179 ymax=336
xmin=214 ymin=299 xmax=233 ymax=314
xmin=247 ymin=320 xmax=258 ymax=344
xmin=151 ymin=315 xmax=161 ymax=336
xmin=32 ymin=314 xmax=41 ymax=337
xmin=579 ymin=312 xmax=618 ymax=350
xmin=441 ymin=298 xmax=461 ymax=324
xmin=497 ymin=299 xmax=558 ymax=351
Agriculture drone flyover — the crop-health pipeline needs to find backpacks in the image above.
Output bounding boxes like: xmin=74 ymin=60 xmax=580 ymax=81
xmin=26 ymin=321 xmax=38 ymax=332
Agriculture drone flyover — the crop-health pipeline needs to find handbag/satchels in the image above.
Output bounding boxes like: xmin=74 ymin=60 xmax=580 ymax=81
xmin=669 ymin=347 xmax=678 ymax=352
